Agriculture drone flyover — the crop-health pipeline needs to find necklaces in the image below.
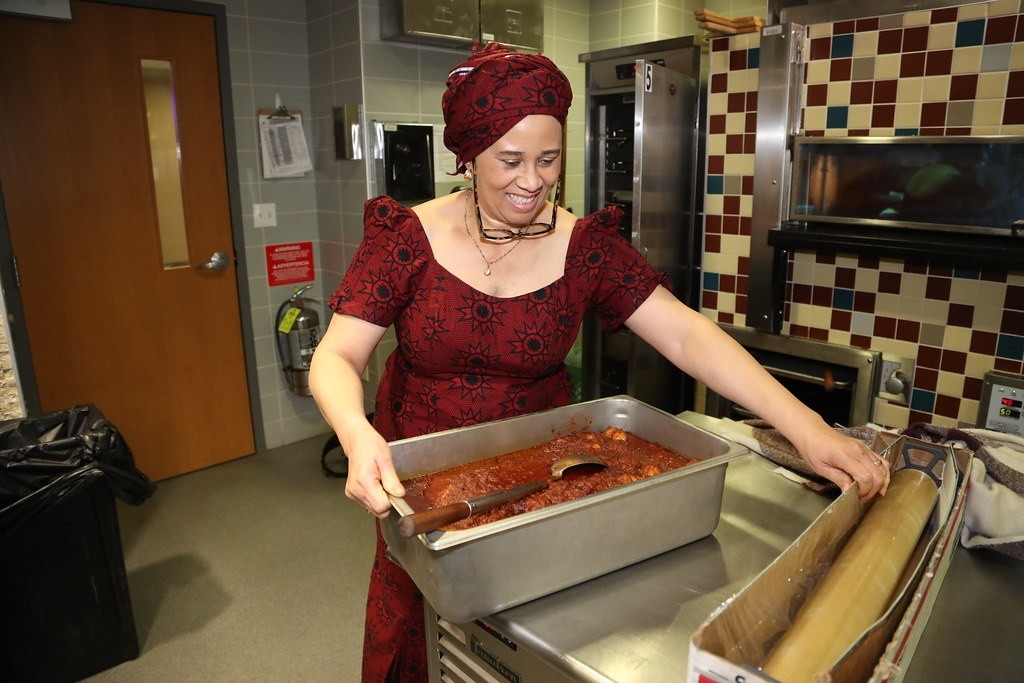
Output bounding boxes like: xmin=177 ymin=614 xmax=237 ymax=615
xmin=464 ymin=187 xmax=534 ymax=276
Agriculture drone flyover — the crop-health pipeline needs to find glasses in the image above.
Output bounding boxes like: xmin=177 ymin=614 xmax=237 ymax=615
xmin=472 ymin=157 xmax=561 ymax=240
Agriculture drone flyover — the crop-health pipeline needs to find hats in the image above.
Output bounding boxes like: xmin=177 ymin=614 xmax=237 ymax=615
xmin=442 ymin=40 xmax=573 ymax=176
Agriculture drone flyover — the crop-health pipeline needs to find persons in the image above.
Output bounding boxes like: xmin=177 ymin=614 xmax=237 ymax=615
xmin=307 ymin=44 xmax=889 ymax=682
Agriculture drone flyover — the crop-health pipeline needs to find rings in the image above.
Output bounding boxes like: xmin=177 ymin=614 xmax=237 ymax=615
xmin=875 ymin=460 xmax=882 ymax=466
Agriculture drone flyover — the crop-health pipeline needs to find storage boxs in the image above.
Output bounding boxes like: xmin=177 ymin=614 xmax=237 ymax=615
xmin=685 ymin=435 xmax=974 ymax=683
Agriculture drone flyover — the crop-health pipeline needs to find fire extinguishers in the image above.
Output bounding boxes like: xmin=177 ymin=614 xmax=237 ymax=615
xmin=275 ymin=284 xmax=323 ymax=397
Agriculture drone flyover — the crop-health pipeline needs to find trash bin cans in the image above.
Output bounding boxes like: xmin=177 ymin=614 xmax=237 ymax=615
xmin=1 ymin=405 xmax=139 ymax=683
